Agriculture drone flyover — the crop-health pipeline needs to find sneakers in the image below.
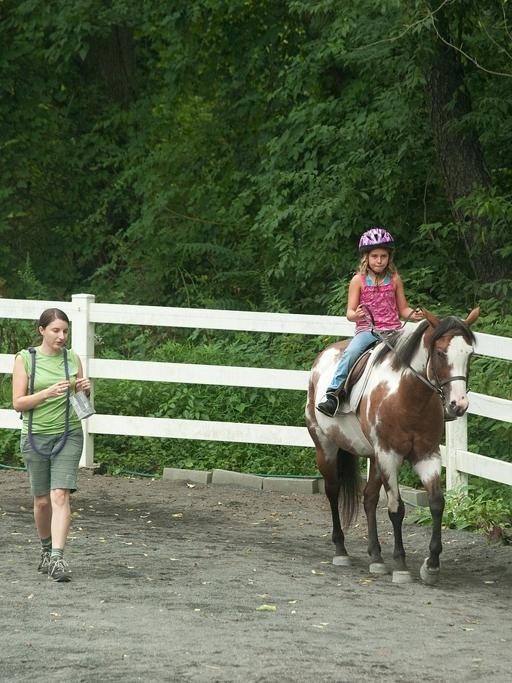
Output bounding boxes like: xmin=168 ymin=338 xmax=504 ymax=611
xmin=318 ymin=399 xmax=337 ymax=415
xmin=38 ymin=553 xmax=73 ymax=582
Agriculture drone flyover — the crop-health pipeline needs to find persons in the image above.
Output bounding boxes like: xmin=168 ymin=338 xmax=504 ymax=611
xmin=12 ymin=308 xmax=91 ymax=582
xmin=316 ymin=228 xmax=424 ymax=419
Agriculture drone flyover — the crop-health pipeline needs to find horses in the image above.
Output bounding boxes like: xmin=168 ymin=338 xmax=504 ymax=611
xmin=303 ymin=305 xmax=481 ymax=586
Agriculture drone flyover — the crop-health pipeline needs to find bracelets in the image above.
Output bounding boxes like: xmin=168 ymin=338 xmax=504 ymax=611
xmin=87 ymin=392 xmax=90 ymax=398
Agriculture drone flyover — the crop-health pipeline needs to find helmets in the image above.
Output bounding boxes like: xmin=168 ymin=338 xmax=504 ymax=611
xmin=359 ymin=229 xmax=394 ymax=250
